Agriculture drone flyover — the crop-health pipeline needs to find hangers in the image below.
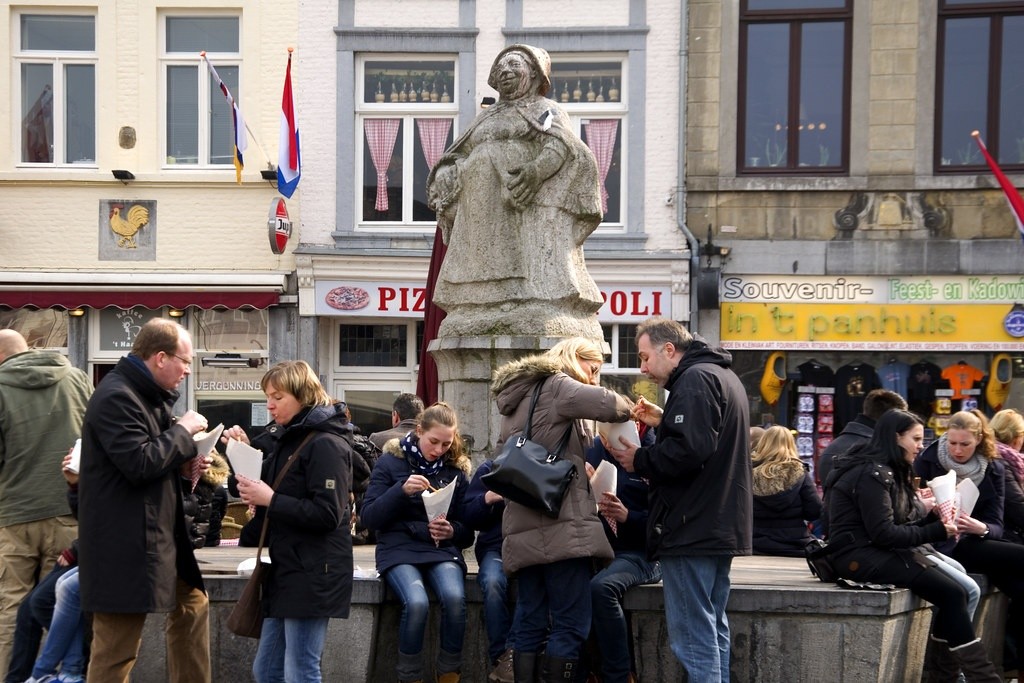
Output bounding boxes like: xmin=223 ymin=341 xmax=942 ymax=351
xmin=797 ymin=350 xmax=987 ymax=386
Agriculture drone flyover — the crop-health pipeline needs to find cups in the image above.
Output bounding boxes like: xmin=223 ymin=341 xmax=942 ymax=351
xmin=66 ymin=439 xmax=82 ymax=477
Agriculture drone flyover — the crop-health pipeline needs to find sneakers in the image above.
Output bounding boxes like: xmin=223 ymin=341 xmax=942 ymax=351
xmin=488 ymin=647 xmax=515 ymax=683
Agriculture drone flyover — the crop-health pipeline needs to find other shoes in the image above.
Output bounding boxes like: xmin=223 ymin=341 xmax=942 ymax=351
xmin=23 ymin=674 xmax=57 ymax=683
xmin=584 ymin=670 xmax=601 ymax=683
xmin=56 ymin=672 xmax=83 ymax=683
xmin=760 ymin=352 xmax=788 ymax=408
xmin=625 ymin=672 xmax=636 ymax=683
xmin=987 ymin=354 xmax=1013 ymax=412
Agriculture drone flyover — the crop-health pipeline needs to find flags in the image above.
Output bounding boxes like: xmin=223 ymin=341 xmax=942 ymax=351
xmin=277 ymin=61 xmax=301 ymax=200
xmin=977 ymin=137 xmax=1024 ymax=244
xmin=208 ymin=66 xmax=248 ymax=187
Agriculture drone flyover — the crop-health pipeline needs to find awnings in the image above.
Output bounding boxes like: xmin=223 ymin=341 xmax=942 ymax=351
xmin=0 ymin=283 xmax=281 ymax=314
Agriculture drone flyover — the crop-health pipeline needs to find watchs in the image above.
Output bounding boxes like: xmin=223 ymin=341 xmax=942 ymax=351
xmin=979 ymin=529 xmax=989 ymax=538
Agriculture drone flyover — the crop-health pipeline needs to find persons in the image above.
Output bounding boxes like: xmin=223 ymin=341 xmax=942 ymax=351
xmin=425 ymin=45 xmax=605 ymax=337
xmin=76 ymin=317 xmax=213 ymax=683
xmin=0 ymin=328 xmax=95 ymax=683
xmin=749 ymin=426 xmax=823 ymax=559
xmin=582 ymin=395 xmax=661 ymax=683
xmin=4 ymin=418 xmax=229 ymax=683
xmin=492 ymin=338 xmax=637 ymax=683
xmin=361 ymin=403 xmax=475 ymax=683
xmin=237 ymin=393 xmax=424 ymax=549
xmin=807 ymin=390 xmax=1003 ymax=683
xmin=914 ymin=407 xmax=1024 ymax=671
xmin=221 ymin=361 xmax=354 ymax=683
xmin=610 ymin=317 xmax=753 ymax=683
xmin=460 ymin=459 xmax=517 ymax=683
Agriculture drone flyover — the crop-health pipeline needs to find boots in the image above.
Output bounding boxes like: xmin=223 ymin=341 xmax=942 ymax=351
xmin=930 ymin=633 xmax=965 ymax=683
xmin=949 ymin=637 xmax=1003 ymax=683
xmin=516 ymin=652 xmax=541 ymax=683
xmin=396 ymin=648 xmax=424 ymax=683
xmin=543 ymin=654 xmax=579 ymax=683
xmin=432 ymin=648 xmax=463 ymax=683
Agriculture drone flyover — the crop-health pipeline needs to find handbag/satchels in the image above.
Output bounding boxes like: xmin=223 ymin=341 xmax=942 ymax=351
xmin=806 ymin=538 xmax=840 ymax=582
xmin=479 ymin=375 xmax=578 ymax=520
xmin=226 ymin=559 xmax=276 ymax=640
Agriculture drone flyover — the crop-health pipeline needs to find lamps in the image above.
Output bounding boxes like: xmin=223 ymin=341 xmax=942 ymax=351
xmin=111 ymin=170 xmax=135 ymax=184
xmin=201 ymin=358 xmax=258 ymax=369
xmin=480 ymin=97 xmax=496 ymax=108
xmin=68 ymin=308 xmax=84 ymax=316
xmin=169 ymin=309 xmax=185 ymax=317
xmin=259 ymin=170 xmax=279 ymax=189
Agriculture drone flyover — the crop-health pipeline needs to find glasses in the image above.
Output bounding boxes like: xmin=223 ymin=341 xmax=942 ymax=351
xmin=585 ymin=359 xmax=600 ymax=378
xmin=167 ymin=352 xmax=192 ymax=370
